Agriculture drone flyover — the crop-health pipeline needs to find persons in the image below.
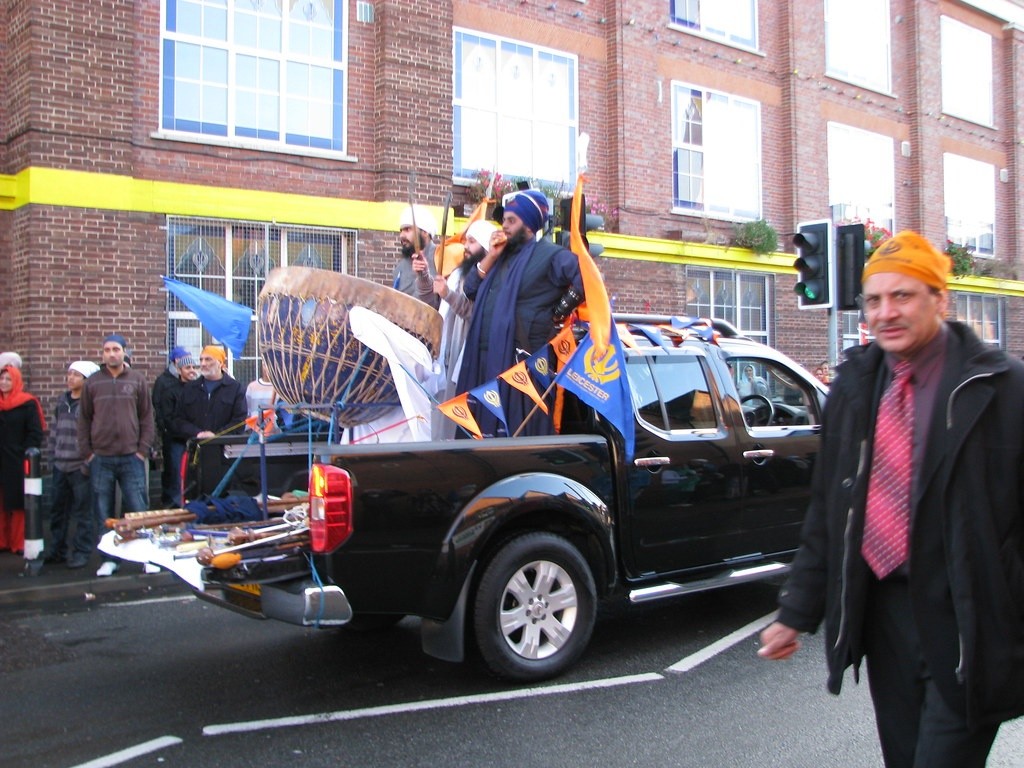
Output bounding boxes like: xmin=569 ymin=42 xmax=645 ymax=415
xmin=783 ymin=361 xmax=830 ymax=406
xmin=151 ymin=346 xmax=248 ymax=510
xmin=727 ymin=362 xmax=767 ymax=397
xmin=78 ymin=335 xmax=162 ymax=578
xmin=246 ymin=361 xmax=281 ymax=417
xmin=0 ymin=352 xmax=47 ymax=557
xmin=757 ymin=230 xmax=1024 ymax=768
xmin=43 ymin=360 xmax=101 ymax=566
xmin=391 ymin=190 xmax=585 ymax=440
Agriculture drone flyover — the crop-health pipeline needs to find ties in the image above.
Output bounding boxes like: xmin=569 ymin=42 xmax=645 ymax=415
xmin=860 ymin=358 xmax=915 ymax=580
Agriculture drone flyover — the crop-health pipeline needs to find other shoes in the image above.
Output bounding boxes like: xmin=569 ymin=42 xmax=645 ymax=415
xmin=96 ymin=561 xmax=120 ymax=577
xmin=0 ymin=548 xmax=8 ymax=552
xmin=65 ymin=557 xmax=89 ymax=569
xmin=25 ymin=561 xmax=64 ymax=577
xmin=144 ymin=563 xmax=161 ymax=574
xmin=15 ymin=550 xmax=24 ymax=555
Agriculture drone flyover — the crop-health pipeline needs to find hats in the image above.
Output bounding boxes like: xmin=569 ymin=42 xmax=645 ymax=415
xmin=124 ymin=347 xmax=133 ymax=368
xmin=400 ymin=205 xmax=436 ymax=238
xmin=862 ymin=229 xmax=952 ymax=288
xmin=503 ymin=189 xmax=549 ymax=235
xmin=201 ymin=344 xmax=226 ymax=363
xmin=466 ymin=220 xmax=507 ymax=257
xmin=170 ymin=345 xmax=191 ymax=362
xmin=103 ymin=335 xmax=126 ymax=350
xmin=178 ymin=357 xmax=196 ymax=368
xmin=67 ymin=360 xmax=100 ymax=379
xmin=1 ymin=352 xmax=23 ymax=372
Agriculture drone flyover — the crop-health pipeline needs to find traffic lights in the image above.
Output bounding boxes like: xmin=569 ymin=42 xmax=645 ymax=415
xmin=792 ymin=223 xmax=830 ymax=305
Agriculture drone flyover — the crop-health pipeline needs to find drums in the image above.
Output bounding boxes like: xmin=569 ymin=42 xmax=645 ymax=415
xmin=258 ymin=264 xmax=445 ymax=432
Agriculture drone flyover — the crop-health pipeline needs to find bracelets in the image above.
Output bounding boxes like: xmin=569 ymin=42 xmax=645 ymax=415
xmin=477 ymin=262 xmax=487 ymax=275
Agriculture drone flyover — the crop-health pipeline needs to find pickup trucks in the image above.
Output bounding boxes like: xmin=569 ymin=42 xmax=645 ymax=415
xmin=98 ymin=316 xmax=834 ymax=686
